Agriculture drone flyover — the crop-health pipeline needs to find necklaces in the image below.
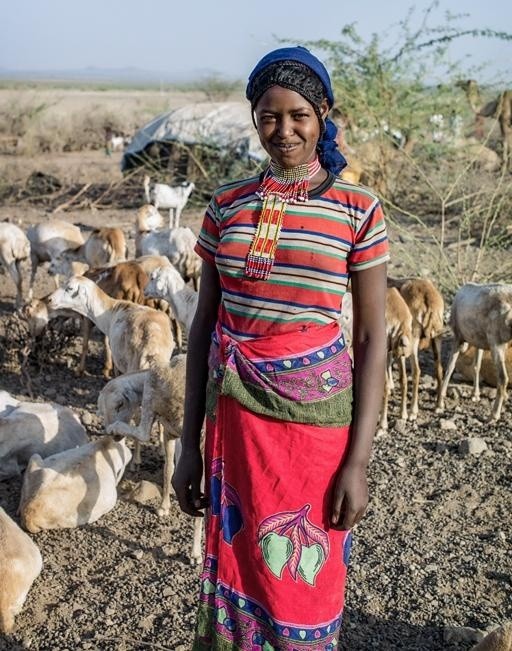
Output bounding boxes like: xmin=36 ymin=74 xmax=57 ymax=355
xmin=255 ymin=155 xmax=324 ymax=203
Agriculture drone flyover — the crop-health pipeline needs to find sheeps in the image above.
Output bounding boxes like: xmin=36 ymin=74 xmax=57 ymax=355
xmin=332 ymin=108 xmax=501 ymax=184
xmin=0 ymin=506 xmax=43 ymax=636
xmin=0 ymin=390 xmax=89 ymax=482
xmin=1 ymin=207 xmax=203 ymax=379
xmin=103 ymin=127 xmax=125 ymax=152
xmin=94 ymin=354 xmax=204 ymax=566
xmin=44 ymin=275 xmax=176 ymax=472
xmin=16 ymin=420 xmax=136 ymax=534
xmin=376 ymin=277 xmax=512 ymax=439
xmin=143 ymin=175 xmax=199 ymax=230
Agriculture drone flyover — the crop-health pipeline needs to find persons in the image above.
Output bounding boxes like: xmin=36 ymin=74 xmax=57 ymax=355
xmin=169 ymin=44 xmax=392 ymax=651
